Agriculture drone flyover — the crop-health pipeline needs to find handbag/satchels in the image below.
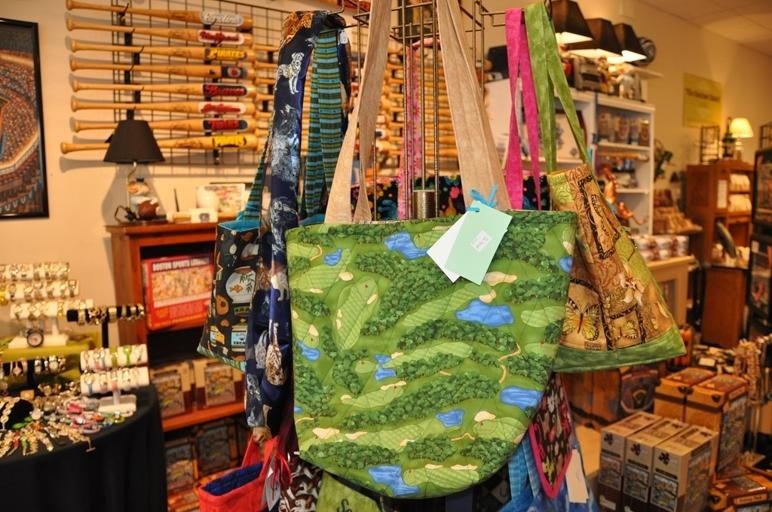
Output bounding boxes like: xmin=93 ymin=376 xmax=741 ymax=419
xmin=195 ymin=0 xmax=686 ymax=511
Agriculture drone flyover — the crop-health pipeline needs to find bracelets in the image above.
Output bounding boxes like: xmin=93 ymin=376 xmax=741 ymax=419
xmin=0 ymin=262 xmax=145 ymax=456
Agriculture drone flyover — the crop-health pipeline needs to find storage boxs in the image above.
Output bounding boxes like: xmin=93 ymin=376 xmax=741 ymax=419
xmin=593 ymin=364 xmax=750 ymax=512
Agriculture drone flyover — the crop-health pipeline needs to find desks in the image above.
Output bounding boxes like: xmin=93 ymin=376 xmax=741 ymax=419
xmin=0 ymin=370 xmax=168 ymax=512
xmin=560 ymin=254 xmax=696 ymax=423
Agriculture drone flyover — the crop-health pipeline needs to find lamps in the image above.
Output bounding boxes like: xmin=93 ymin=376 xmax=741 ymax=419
xmin=547 ymin=0 xmax=657 ymax=64
xmin=721 ymin=117 xmax=754 ymax=162
xmin=103 ymin=119 xmax=168 ymax=225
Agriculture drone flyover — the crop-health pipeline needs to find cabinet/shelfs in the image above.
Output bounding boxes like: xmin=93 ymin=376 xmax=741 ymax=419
xmin=688 ymin=163 xmax=754 ymax=349
xmin=750 ymin=148 xmax=772 ymax=336
xmin=106 ymin=217 xmax=259 ymax=512
xmin=482 ymin=77 xmax=654 ymax=258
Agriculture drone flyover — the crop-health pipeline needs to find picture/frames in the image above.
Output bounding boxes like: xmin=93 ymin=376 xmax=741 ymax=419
xmin=0 ymin=15 xmax=50 ymax=221
xmin=700 ymin=125 xmax=720 ymax=163
